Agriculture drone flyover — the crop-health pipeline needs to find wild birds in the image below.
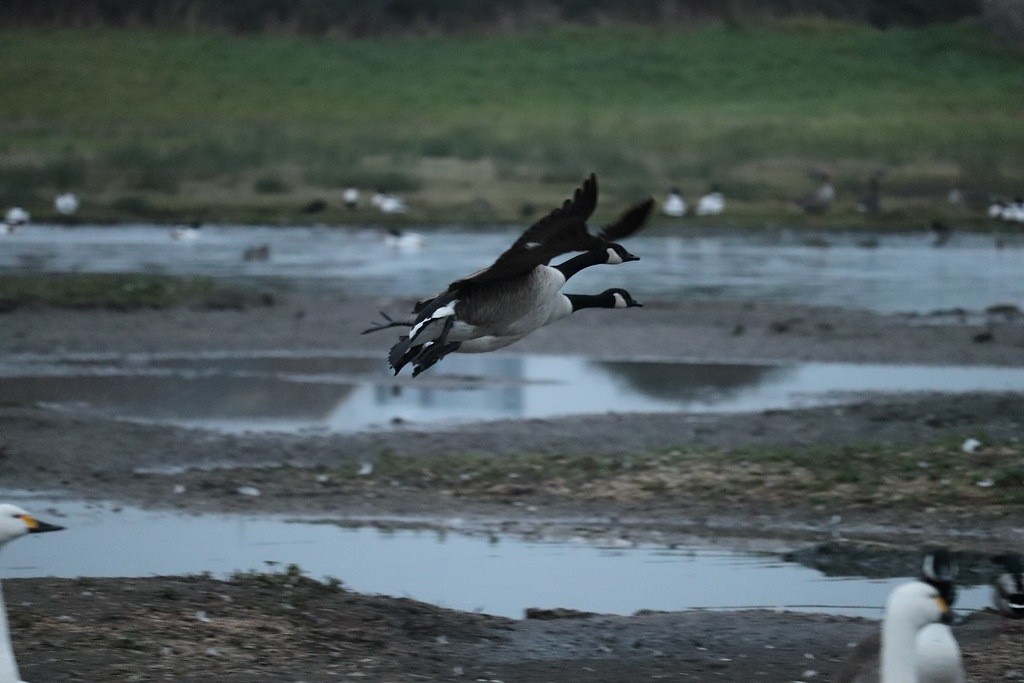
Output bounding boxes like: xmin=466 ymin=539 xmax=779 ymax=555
xmin=662 ymin=182 xmax=727 ymax=219
xmin=359 ymin=172 xmax=656 ymax=380
xmin=1 ymin=504 xmax=70 ymax=683
xmin=3 ymin=180 xmax=84 ymax=227
xmin=343 ymin=175 xmax=411 ymax=216
xmin=878 ymin=544 xmax=968 ymax=683
xmin=985 ymin=195 xmax=1024 ymax=224
